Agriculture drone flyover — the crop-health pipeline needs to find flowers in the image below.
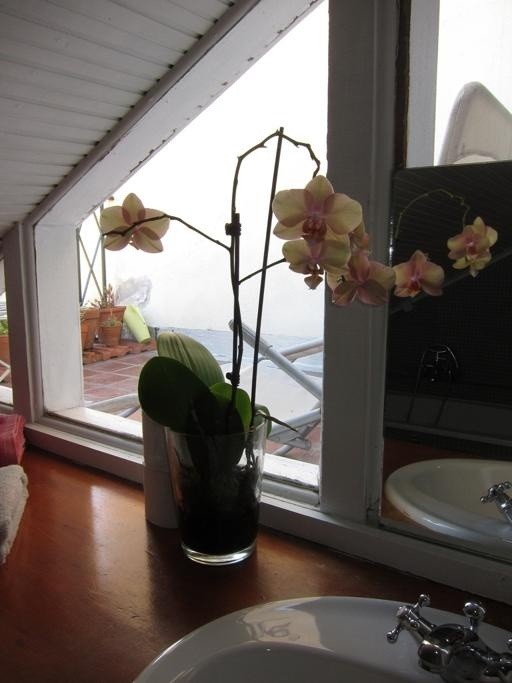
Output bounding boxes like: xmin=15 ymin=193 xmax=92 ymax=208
xmin=96 ymin=126 xmax=497 ymax=539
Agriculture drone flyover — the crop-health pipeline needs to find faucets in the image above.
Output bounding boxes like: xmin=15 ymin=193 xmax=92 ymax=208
xmin=407 ymin=339 xmax=461 ymax=421
xmin=418 ymin=620 xmax=497 ymax=681
xmin=480 ymin=481 xmax=512 ymax=518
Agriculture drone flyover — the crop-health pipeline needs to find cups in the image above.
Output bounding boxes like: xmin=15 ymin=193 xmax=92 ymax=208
xmin=166 ymin=418 xmax=264 ymax=565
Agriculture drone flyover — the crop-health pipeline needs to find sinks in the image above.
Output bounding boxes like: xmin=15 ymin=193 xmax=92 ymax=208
xmin=385 ymin=456 xmax=512 ymax=553
xmin=131 ymin=593 xmax=511 ymax=683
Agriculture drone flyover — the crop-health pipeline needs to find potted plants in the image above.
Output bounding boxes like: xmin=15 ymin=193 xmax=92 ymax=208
xmin=79 ymin=284 xmax=127 ymax=352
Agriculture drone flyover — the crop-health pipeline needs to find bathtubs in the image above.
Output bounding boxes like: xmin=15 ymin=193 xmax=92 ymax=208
xmin=384 ymin=392 xmax=511 ymax=446
